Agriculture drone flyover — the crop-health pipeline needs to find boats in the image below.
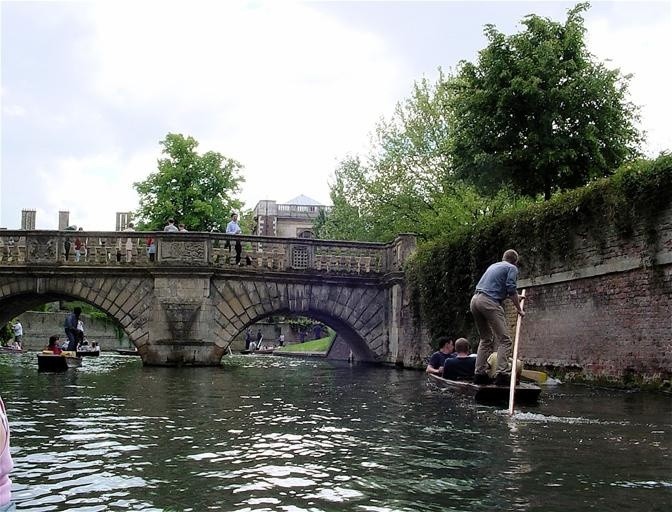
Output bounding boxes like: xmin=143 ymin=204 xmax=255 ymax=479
xmin=115 ymin=348 xmax=139 ymax=355
xmin=0 ymin=345 xmax=28 ymax=354
xmin=223 ymin=350 xmax=228 ymax=355
xmin=427 ymin=373 xmax=541 ymax=405
xmin=37 ymin=353 xmax=83 ymax=369
xmin=241 ymin=347 xmax=278 ymax=354
xmin=62 ymin=348 xmax=99 ymax=357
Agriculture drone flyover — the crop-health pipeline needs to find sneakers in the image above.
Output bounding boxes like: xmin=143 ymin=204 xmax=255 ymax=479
xmin=474 ymin=374 xmax=490 ymax=384
xmin=496 ymin=374 xmax=506 ymax=384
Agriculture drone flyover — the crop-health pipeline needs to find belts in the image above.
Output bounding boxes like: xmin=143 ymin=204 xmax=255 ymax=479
xmin=474 ymin=291 xmax=489 ymax=296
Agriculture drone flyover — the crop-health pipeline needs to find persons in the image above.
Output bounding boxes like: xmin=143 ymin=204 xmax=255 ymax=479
xmin=226 ymin=213 xmax=245 ymax=266
xmin=164 ymin=218 xmax=179 ymax=231
xmin=119 ymin=222 xmax=140 ymax=262
xmin=424 ymin=335 xmax=523 ymax=384
xmin=6 ymin=305 xmax=101 ymax=357
xmin=177 ymin=223 xmax=188 ymax=231
xmin=74 ymin=227 xmax=84 ymax=262
xmin=0 ymin=394 xmax=17 ymax=511
xmin=243 ymin=323 xmax=323 ymax=351
xmin=146 ymin=230 xmax=158 ymax=261
xmin=469 ymin=247 xmax=526 ymax=386
